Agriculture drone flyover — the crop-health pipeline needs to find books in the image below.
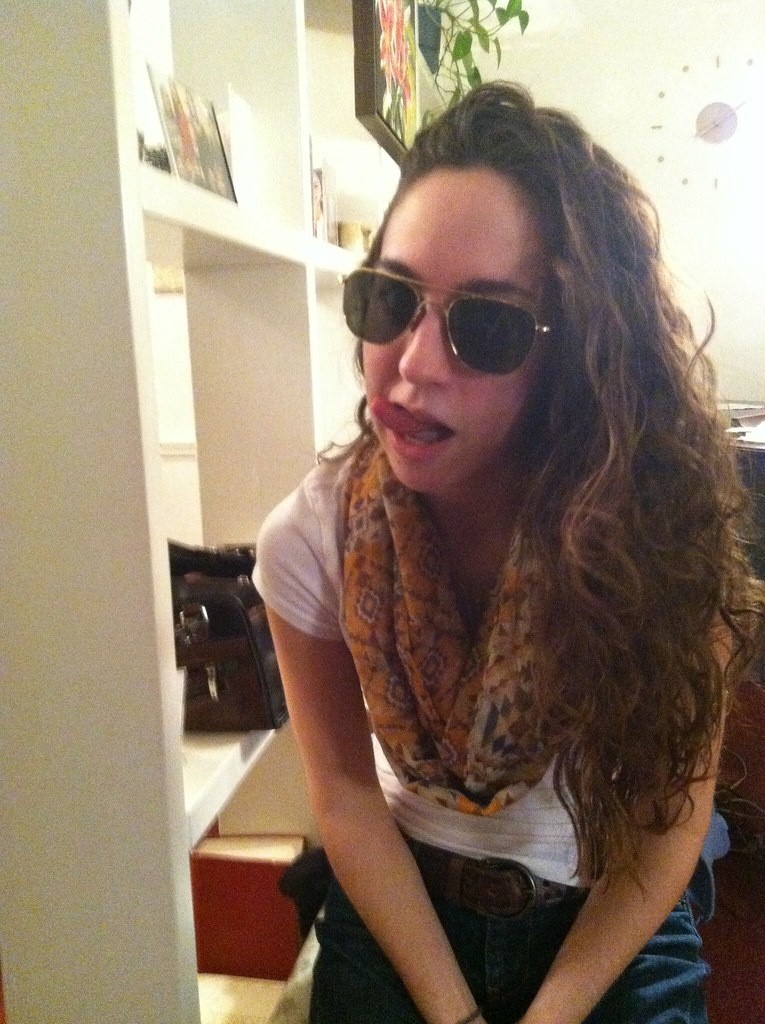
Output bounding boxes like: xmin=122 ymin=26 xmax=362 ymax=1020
xmin=192 ymin=834 xmax=307 ymax=981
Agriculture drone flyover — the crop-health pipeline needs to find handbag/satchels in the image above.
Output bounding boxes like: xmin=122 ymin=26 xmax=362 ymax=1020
xmin=165 ymin=539 xmax=288 ymax=731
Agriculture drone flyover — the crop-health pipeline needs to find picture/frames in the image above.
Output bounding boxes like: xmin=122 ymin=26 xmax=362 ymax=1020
xmin=147 ymin=63 xmax=241 ymax=203
xmin=353 ymin=1 xmax=420 ymax=164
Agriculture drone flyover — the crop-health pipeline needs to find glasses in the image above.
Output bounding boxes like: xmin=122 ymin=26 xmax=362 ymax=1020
xmin=339 ymin=268 xmax=552 ymax=375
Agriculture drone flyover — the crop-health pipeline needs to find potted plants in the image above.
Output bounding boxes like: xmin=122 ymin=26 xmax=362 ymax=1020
xmin=418 ymin=0 xmax=529 ymax=127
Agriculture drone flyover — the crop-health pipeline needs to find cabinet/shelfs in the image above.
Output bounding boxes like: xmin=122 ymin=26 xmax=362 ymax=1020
xmin=1 ymin=0 xmax=430 ymax=1024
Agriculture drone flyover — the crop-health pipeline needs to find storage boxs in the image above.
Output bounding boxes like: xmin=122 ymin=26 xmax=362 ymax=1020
xmin=193 ymin=834 xmax=314 ymax=982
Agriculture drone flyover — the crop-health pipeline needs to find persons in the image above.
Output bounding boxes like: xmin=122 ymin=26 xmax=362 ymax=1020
xmin=160 ymin=78 xmax=237 ymax=203
xmin=252 ymin=83 xmax=765 ymax=1024
xmin=313 ymin=168 xmax=324 ymax=239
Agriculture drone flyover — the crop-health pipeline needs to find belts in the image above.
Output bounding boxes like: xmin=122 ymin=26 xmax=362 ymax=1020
xmin=412 ymin=847 xmax=587 ymax=921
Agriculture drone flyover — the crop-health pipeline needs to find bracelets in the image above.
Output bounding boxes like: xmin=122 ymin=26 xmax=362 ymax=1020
xmin=459 ymin=1006 xmax=482 ymax=1024
